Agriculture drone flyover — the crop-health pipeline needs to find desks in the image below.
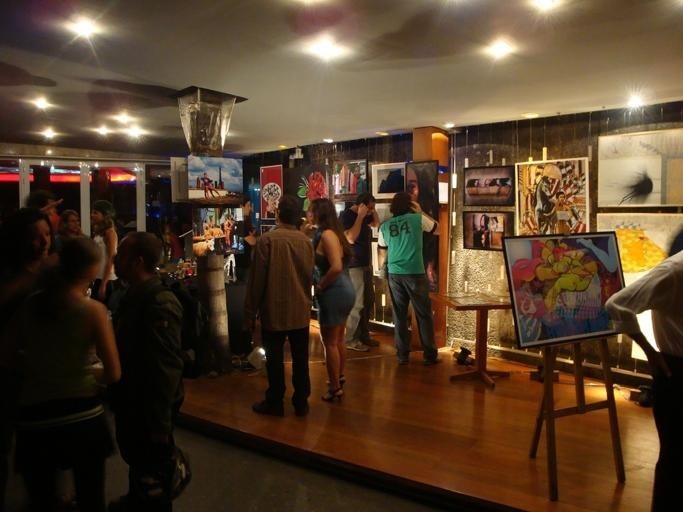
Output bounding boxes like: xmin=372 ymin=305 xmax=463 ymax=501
xmin=428 ymin=289 xmax=515 ymax=387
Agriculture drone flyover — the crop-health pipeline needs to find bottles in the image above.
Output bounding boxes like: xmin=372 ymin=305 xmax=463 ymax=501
xmin=175 ymin=255 xmax=197 ymax=280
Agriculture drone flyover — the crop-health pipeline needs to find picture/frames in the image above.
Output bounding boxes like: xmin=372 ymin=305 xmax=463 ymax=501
xmin=462 ymin=165 xmax=515 ymax=207
xmin=514 ymin=156 xmax=591 ymax=237
xmin=369 ymin=162 xmax=407 ymax=199
xmin=597 ymin=128 xmax=683 ymax=208
xmin=596 ymin=213 xmax=683 ymax=288
xmin=461 ymin=211 xmax=514 ymax=251
xmin=404 ymin=158 xmax=439 ymax=293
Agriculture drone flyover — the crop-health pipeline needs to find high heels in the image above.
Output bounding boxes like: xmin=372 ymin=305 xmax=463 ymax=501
xmin=320 ymin=388 xmax=345 ymax=403
xmin=325 ymin=375 xmax=346 ymax=387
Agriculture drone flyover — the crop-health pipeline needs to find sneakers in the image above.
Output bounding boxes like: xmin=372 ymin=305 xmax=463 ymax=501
xmin=395 ymin=352 xmax=409 ymax=366
xmin=291 ymin=404 xmax=310 ymax=416
xmin=345 ymin=338 xmax=380 ymax=352
xmin=252 ymin=399 xmax=285 ymax=417
xmin=423 ymin=354 xmax=443 ymax=366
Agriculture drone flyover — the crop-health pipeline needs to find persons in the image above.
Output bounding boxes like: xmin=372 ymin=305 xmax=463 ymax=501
xmin=475 ymin=215 xmax=499 ymax=248
xmin=251 ymin=190 xmax=316 ymax=416
xmin=233 ymin=198 xmax=258 ymax=281
xmin=378 ymin=191 xmax=441 ymax=366
xmin=543 ymin=192 xmax=573 ymax=234
xmin=338 ymin=194 xmax=381 ymax=351
xmin=352 ymin=166 xmax=364 ymax=193
xmin=298 ymin=198 xmax=355 ymax=403
xmin=605 ymin=250 xmax=682 ymax=512
xmin=1 ymin=200 xmax=190 ymax=512
